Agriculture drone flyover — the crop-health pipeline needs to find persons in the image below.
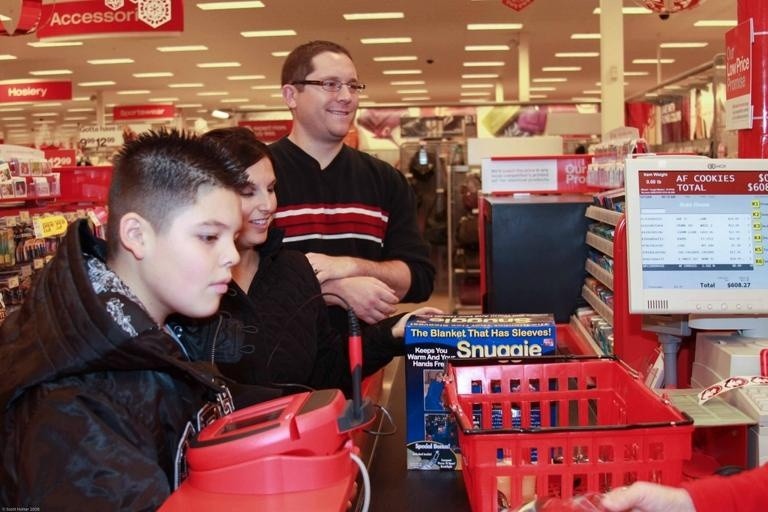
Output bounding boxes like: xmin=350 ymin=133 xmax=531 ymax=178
xmin=168 ymin=125 xmax=444 ymax=398
xmin=601 ymin=461 xmax=768 ymax=512
xmin=0 ymin=125 xmax=253 ymax=512
xmin=263 ymin=41 xmax=436 ymax=325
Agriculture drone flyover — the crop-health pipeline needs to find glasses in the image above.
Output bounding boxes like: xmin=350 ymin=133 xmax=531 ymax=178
xmin=290 ymin=79 xmax=366 ymax=94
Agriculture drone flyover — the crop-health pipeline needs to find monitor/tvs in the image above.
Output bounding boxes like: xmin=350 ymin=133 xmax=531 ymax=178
xmin=625 ymin=159 xmax=768 ymax=331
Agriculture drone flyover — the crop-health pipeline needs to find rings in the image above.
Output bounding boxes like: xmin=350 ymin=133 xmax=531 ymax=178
xmin=314 ymin=266 xmax=318 ymax=274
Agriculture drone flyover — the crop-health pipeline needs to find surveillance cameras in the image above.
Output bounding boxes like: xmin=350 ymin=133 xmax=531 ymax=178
xmin=427 ymin=60 xmax=433 ymax=64
xmin=659 ymin=14 xmax=669 ymax=20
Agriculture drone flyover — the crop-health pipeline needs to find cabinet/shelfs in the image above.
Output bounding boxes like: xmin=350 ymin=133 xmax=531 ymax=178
xmin=0 ymin=143 xmax=109 ymax=319
xmin=564 ymin=201 xmax=694 ymax=388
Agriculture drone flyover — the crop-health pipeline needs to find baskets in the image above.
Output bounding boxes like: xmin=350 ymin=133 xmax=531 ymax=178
xmin=444 ymin=355 xmax=694 ymax=512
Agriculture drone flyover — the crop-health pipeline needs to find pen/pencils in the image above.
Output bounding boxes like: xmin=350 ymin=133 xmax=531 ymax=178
xmin=615 ymin=203 xmax=622 ymax=212
xmin=592 ymin=192 xmax=614 ymax=209
